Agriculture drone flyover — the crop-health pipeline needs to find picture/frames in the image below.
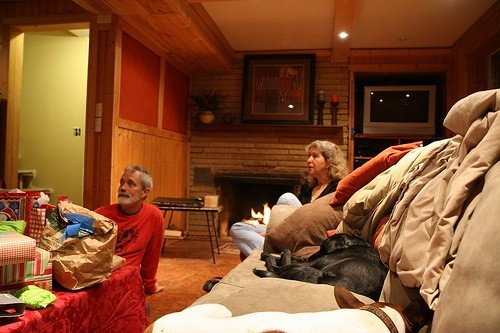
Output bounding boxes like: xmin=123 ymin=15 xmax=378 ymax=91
xmin=240 ymin=52 xmax=316 ymax=126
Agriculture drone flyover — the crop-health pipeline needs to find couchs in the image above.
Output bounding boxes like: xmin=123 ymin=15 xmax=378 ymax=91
xmin=188 ymin=204 xmax=500 ymax=333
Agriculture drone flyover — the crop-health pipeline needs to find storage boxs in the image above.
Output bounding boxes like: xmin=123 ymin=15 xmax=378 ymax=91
xmin=0 ymin=230 xmax=53 ymax=292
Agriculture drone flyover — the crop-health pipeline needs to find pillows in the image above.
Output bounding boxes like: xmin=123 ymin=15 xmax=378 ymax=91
xmin=265 ymin=191 xmax=345 ymax=255
xmin=330 ymin=141 xmax=424 ymax=206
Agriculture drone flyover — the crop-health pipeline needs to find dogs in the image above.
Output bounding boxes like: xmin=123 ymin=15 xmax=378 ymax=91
xmin=144 ymin=285 xmax=410 ymax=333
xmin=253 ymin=233 xmax=389 ymax=302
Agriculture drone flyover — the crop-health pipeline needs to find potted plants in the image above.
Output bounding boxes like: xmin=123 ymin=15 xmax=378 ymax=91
xmin=186 ymin=90 xmax=228 ymax=124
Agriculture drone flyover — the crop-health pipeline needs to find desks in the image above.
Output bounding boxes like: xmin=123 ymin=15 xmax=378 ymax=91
xmin=150 ymin=204 xmax=223 ymax=264
xmin=0 ymin=263 xmax=150 ymax=333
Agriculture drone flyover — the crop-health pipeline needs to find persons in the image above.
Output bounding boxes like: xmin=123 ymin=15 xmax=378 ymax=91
xmin=229 ymin=140 xmax=348 ymax=257
xmin=92 ymin=165 xmax=164 ymax=294
